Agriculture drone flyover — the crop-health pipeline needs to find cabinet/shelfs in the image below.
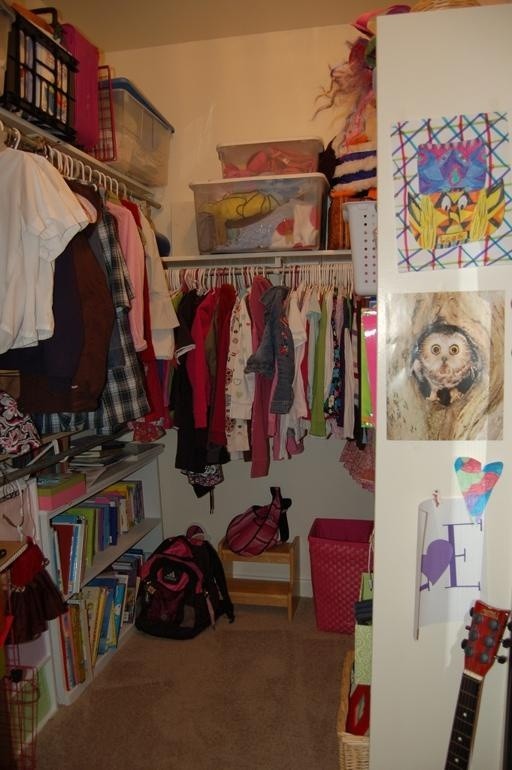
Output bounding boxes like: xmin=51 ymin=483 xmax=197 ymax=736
xmin=217 ymin=535 xmax=301 ymax=623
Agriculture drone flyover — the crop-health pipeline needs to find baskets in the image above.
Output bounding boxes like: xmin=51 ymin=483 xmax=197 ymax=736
xmin=336 ymin=650 xmax=369 ymax=770
xmin=328 ymin=197 xmax=375 ymax=251
xmin=7 ymin=15 xmax=76 ymax=144
xmin=340 ymin=195 xmax=378 ymax=297
xmin=309 ymin=516 xmax=373 ymax=635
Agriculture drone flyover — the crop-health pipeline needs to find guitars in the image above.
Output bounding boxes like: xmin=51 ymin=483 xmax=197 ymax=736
xmin=444 ymin=600 xmax=511 ymax=770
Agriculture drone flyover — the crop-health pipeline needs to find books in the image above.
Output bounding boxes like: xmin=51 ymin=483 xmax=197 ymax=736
xmin=1 ymin=540 xmax=28 ymax=574
xmin=37 ymin=436 xmax=151 ymax=694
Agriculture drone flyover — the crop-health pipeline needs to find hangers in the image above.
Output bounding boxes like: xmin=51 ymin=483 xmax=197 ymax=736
xmin=0 ymin=119 xmax=141 ymax=200
xmin=159 ymin=262 xmax=356 ymax=300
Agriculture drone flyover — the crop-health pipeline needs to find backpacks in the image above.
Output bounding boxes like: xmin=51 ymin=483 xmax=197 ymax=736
xmin=132 ymin=528 xmax=234 ymax=640
xmin=225 ymin=486 xmax=291 ymax=557
